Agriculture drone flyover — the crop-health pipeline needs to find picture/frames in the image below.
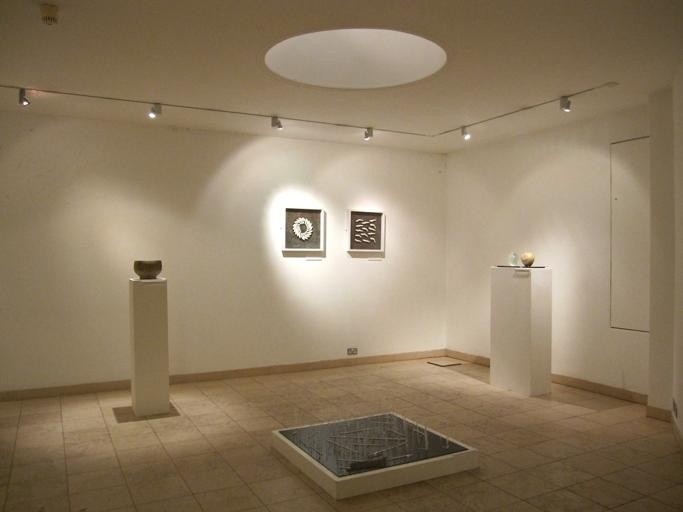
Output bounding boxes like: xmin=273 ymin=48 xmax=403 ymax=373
xmin=282 ymin=207 xmax=385 ymax=252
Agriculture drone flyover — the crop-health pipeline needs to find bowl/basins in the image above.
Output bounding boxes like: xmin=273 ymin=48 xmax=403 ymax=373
xmin=134 ymin=260 xmax=162 ymax=280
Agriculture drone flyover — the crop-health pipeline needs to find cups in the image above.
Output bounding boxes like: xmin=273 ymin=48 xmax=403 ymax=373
xmin=520 ymin=253 xmax=534 ymax=267
